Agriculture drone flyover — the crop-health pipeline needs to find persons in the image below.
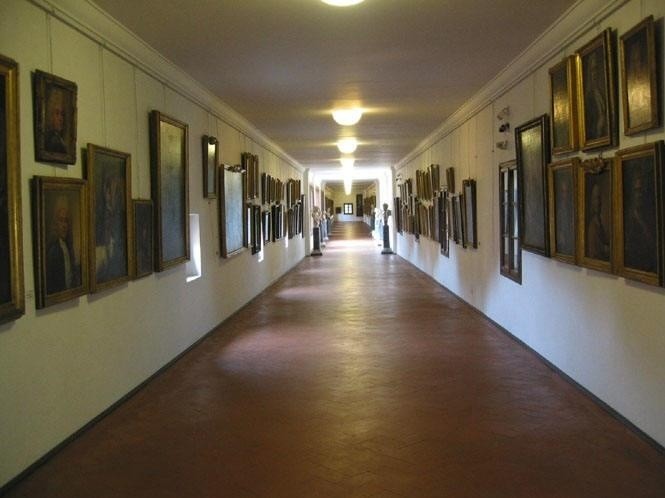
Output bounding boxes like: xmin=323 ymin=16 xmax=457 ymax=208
xmin=380 ymin=202 xmax=392 ymax=223
xmin=312 ymin=206 xmax=322 ymax=225
xmin=585 ymin=180 xmax=612 ymax=261
xmin=321 ymin=210 xmax=327 ymax=221
xmin=624 ymin=175 xmax=658 ymax=273
xmin=40 ymin=86 xmax=75 ymax=154
xmin=555 ymin=182 xmax=573 ymax=256
xmin=581 ymin=52 xmax=610 ymax=141
xmin=45 ymin=191 xmax=83 ymax=295
xmin=325 ymin=206 xmax=332 ymax=219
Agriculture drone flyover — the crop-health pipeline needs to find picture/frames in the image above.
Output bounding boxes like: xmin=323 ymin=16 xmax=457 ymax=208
xmin=202 ymin=134 xmax=307 ymax=259
xmin=514 ymin=115 xmax=549 ymax=258
xmin=28 ymin=67 xmax=193 ymax=309
xmin=614 ymin=138 xmax=664 ymax=286
xmin=580 ymin=155 xmax=615 ymax=276
xmin=572 ymin=27 xmax=618 ymax=152
xmin=618 ymin=16 xmax=663 ymax=136
xmin=393 ymin=165 xmax=478 ymax=256
xmin=548 ymin=158 xmax=579 ymax=267
xmin=548 ymin=54 xmax=578 ymax=155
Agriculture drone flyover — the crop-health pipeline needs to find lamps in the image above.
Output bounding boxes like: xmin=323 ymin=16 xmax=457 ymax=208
xmin=498 ymin=121 xmax=507 ymax=132
xmin=495 ymin=139 xmax=506 ymax=151
xmin=496 ymin=107 xmax=508 ymax=119
